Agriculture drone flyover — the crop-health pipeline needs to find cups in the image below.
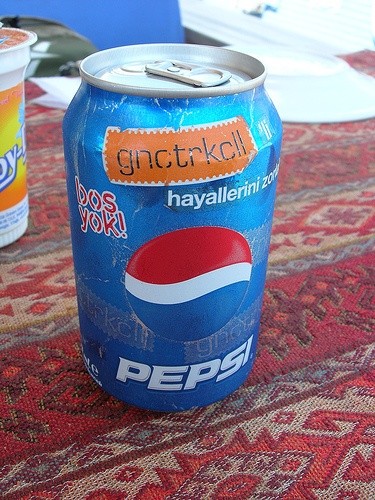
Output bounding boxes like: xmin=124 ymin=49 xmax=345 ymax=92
xmin=0 ymin=23 xmax=39 ymax=248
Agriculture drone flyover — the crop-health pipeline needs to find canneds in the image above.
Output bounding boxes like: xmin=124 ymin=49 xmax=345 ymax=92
xmin=62 ymin=43 xmax=282 ymax=413
